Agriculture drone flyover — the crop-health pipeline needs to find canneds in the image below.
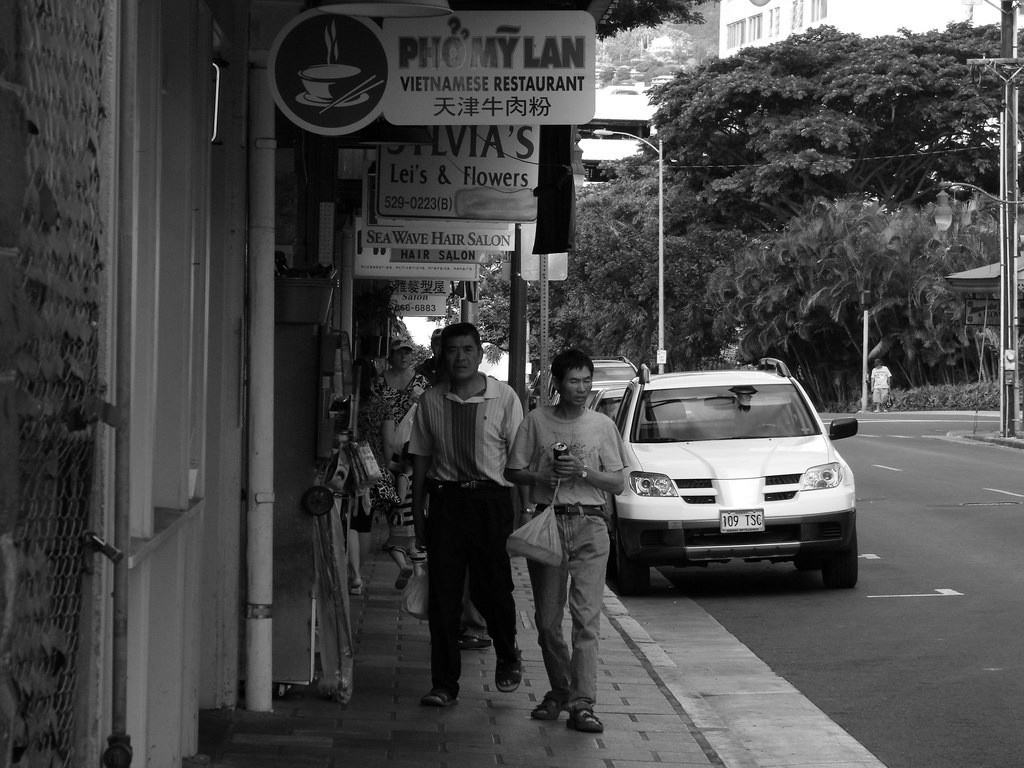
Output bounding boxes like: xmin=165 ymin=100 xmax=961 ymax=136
xmin=553 ymin=441 xmax=569 ymax=460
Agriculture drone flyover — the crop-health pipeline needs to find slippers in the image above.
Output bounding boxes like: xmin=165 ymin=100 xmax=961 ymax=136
xmin=456 ymin=635 xmax=492 ymax=648
xmin=350 ymin=584 xmax=362 ymax=595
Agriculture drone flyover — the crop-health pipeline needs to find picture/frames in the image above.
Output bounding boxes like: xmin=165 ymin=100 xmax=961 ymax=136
xmin=275 ymin=140 xmax=306 ymax=270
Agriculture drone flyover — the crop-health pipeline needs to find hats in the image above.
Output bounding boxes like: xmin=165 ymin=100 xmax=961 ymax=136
xmin=391 ymin=337 xmax=414 ymax=351
xmin=431 ymin=329 xmax=443 ymax=342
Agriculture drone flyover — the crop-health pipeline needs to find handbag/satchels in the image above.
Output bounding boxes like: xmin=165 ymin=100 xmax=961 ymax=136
xmin=401 ymin=556 xmax=429 ymax=620
xmin=506 ymin=477 xmax=563 ymax=567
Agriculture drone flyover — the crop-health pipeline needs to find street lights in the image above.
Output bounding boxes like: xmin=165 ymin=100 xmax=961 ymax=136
xmin=594 ymin=127 xmax=668 ymax=377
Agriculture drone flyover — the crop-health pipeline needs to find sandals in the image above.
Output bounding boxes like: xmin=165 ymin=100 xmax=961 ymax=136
xmin=421 ymin=688 xmax=459 ymax=705
xmin=495 ymin=639 xmax=523 ymax=692
xmin=530 ymin=690 xmax=570 ymax=720
xmin=566 ymin=708 xmax=604 ymax=732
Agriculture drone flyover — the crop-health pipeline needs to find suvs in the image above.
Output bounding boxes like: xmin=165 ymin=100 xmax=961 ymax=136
xmin=530 ymin=354 xmax=693 ymax=441
xmin=608 ymin=357 xmax=858 ymax=598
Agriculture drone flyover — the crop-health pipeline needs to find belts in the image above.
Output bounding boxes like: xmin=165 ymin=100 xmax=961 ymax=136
xmin=436 ymin=480 xmax=505 ymax=491
xmin=536 ymin=504 xmax=610 ymax=520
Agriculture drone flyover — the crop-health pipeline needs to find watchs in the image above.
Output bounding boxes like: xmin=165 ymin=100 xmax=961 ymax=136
xmin=578 ymin=464 xmax=588 ymax=479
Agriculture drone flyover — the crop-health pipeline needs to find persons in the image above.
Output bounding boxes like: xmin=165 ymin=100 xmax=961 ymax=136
xmin=504 ymin=347 xmax=629 ymax=734
xmin=348 ymin=327 xmax=493 ymax=650
xmin=871 ymin=359 xmax=892 ymax=412
xmin=409 ymin=324 xmax=525 ymax=706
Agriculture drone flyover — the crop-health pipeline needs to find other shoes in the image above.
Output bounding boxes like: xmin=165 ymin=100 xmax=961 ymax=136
xmin=395 ymin=566 xmax=413 ymax=589
